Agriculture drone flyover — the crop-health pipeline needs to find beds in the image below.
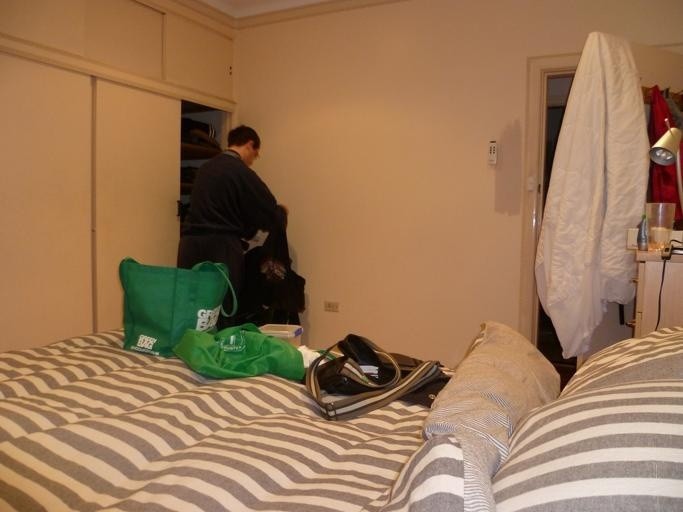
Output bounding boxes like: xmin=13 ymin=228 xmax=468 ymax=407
xmin=0 ymin=327 xmax=683 ymax=509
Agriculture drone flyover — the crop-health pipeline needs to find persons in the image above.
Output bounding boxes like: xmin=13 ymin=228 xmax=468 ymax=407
xmin=177 ymin=124 xmax=287 ymax=329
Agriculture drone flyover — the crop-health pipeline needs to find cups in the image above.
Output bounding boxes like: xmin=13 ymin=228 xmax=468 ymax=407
xmin=645 ymin=202 xmax=676 ymax=257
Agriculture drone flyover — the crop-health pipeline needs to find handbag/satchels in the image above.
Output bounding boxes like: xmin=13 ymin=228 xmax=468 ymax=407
xmin=173 ymin=322 xmax=306 ymax=385
xmin=119 ymin=256 xmax=230 ymax=360
xmin=304 ymin=333 xmax=451 ymax=421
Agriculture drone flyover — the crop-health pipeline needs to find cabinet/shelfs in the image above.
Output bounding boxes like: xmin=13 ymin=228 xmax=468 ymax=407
xmin=3 ymin=2 xmax=238 ymax=352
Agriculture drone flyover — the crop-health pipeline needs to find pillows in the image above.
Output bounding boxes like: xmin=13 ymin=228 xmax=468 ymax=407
xmin=559 ymin=324 xmax=683 ymax=397
xmin=490 ymin=380 xmax=683 ymax=509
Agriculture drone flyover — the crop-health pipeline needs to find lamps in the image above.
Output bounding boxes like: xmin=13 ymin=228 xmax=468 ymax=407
xmin=649 ymin=117 xmax=683 ymax=213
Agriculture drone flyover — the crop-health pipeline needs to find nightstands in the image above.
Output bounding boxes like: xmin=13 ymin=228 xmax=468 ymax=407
xmin=625 ymin=241 xmax=683 ymax=336
